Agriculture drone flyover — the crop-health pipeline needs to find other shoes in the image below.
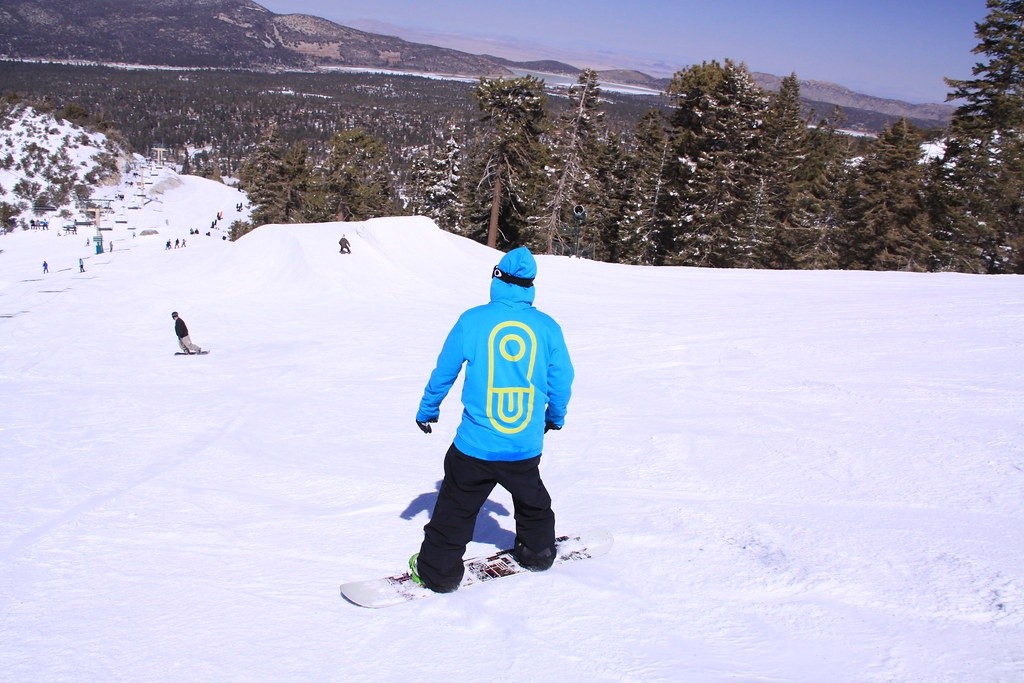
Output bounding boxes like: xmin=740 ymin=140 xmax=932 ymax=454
xmin=409 ymin=553 xmax=424 ymax=585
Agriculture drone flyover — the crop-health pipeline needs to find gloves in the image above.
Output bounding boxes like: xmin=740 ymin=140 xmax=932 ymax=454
xmin=416 ymin=418 xmax=437 ymax=433
xmin=544 ymin=420 xmax=560 ymax=434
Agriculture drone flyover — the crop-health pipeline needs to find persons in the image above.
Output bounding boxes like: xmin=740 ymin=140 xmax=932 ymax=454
xmin=338 ymin=234 xmax=351 ymax=253
xmin=109 ymin=241 xmax=113 ymax=252
xmin=42 ymin=261 xmax=48 ymax=274
xmin=65 ymin=224 xmax=77 ymax=235
xmin=30 ymin=218 xmax=49 ymax=230
xmin=79 ymin=258 xmax=85 ymax=272
xmin=165 ymin=238 xmax=186 ymax=250
xmin=172 ymin=312 xmax=201 ymax=355
xmin=408 ymin=245 xmax=574 ymax=594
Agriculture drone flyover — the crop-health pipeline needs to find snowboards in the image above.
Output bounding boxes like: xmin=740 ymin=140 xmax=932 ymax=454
xmin=339 ymin=250 xmax=351 ymax=254
xmin=339 ymin=525 xmax=615 ymax=608
xmin=174 ymin=350 xmax=210 ymax=355
